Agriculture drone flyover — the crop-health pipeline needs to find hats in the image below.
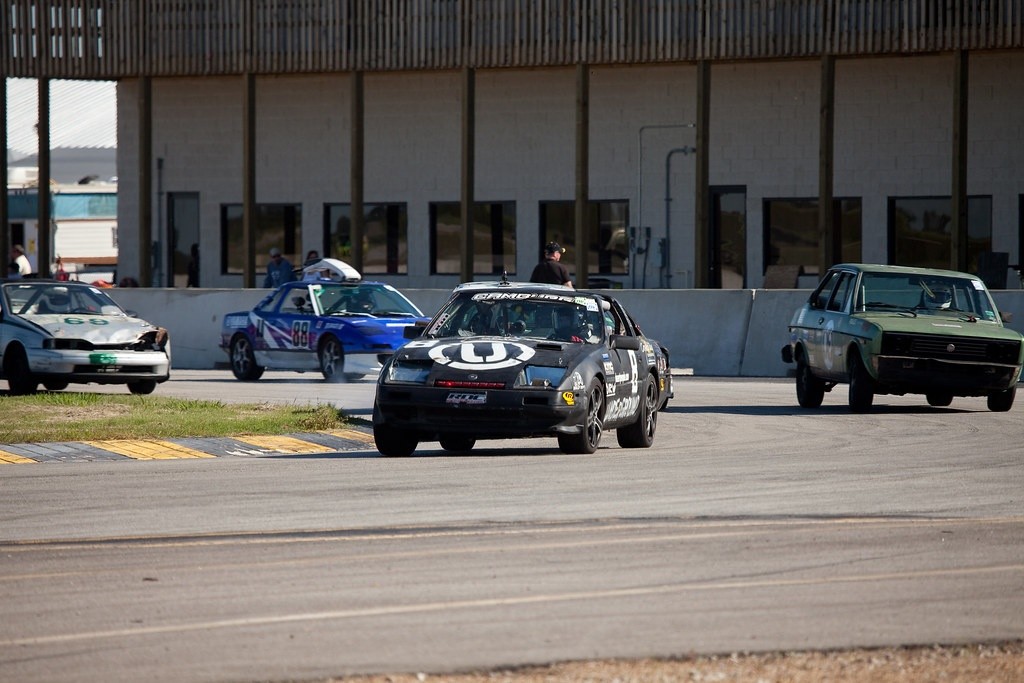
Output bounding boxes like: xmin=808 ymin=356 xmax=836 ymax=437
xmin=545 ymin=242 xmax=565 ymax=256
xmin=12 ymin=245 xmax=25 ymax=254
xmin=269 ymin=248 xmax=280 ymax=256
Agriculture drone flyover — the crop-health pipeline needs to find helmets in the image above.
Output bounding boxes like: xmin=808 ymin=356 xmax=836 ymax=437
xmin=923 ymin=283 xmax=952 ymax=310
xmin=44 ymin=287 xmax=71 ymax=312
xmin=350 ymin=290 xmax=376 ymax=312
xmin=551 ymin=304 xmax=583 ymax=337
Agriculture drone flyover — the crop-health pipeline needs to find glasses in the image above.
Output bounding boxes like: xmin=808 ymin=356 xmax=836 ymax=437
xmin=308 ymin=255 xmax=318 ymax=259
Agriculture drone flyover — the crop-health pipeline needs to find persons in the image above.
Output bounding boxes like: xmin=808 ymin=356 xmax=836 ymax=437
xmin=546 ymin=303 xmax=593 ymax=344
xmin=186 ymin=243 xmax=199 ymax=288
xmin=529 ymin=242 xmax=572 ymax=290
xmin=10 ymin=245 xmax=31 ymax=277
xmin=265 ymin=248 xmax=339 ymax=288
xmin=43 ymin=287 xmax=69 ymax=312
xmin=347 ymin=288 xmax=377 ymax=313
xmin=924 ymin=280 xmax=953 ymax=312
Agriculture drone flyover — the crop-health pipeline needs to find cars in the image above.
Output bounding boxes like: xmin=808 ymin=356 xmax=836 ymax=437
xmin=216 ymin=253 xmax=435 ymax=383
xmin=780 ymin=261 xmax=1023 ymax=414
xmin=369 ymin=276 xmax=675 ymax=457
xmin=0 ymin=275 xmax=172 ymax=396
